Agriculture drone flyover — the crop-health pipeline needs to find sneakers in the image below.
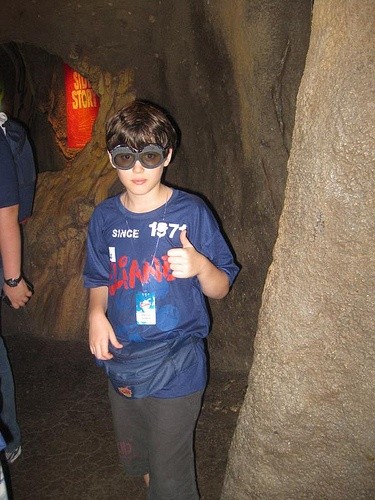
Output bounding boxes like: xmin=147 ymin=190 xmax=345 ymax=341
xmin=0 ymin=445 xmax=22 ymax=480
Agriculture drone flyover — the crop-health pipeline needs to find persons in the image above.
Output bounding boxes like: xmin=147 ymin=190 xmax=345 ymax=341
xmin=83 ymin=101 xmax=240 ymax=500
xmin=0 ymin=111 xmax=34 ymax=463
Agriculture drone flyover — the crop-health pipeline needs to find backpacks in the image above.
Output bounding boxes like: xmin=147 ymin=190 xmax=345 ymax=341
xmin=0 ymin=118 xmax=36 ymax=222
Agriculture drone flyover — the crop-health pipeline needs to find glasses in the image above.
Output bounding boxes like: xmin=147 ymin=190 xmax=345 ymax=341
xmin=109 ymin=144 xmax=169 ymax=171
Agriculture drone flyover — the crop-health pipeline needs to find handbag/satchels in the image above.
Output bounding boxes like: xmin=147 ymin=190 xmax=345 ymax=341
xmin=103 ymin=329 xmax=208 ymax=399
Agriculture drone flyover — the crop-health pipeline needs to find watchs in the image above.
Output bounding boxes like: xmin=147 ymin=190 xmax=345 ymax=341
xmin=4 ymin=273 xmax=22 ymax=287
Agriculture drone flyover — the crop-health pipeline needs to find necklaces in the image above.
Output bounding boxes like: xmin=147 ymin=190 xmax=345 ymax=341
xmin=124 ymin=190 xmax=168 ymax=293
